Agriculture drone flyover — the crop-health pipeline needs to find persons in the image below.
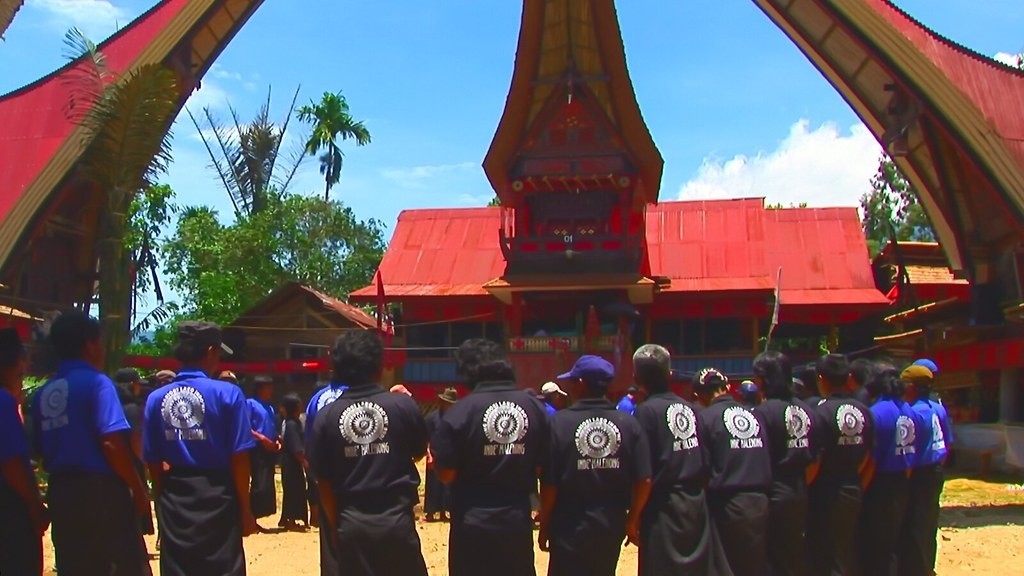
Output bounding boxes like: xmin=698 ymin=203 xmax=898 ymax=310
xmin=0 ymin=311 xmax=950 ymax=576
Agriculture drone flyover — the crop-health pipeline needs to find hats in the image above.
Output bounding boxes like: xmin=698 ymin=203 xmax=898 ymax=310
xmin=912 ymin=358 xmax=938 ymax=374
xmin=389 ymin=383 xmax=412 ymax=397
xmin=523 ymin=388 xmax=545 ymax=400
xmin=690 ymin=367 xmax=729 ymax=401
xmin=437 ymin=387 xmax=457 ymax=404
xmin=557 ymin=355 xmax=616 ymax=384
xmin=542 ymin=381 xmax=568 ymax=396
xmin=735 ymin=379 xmax=759 ymax=396
xmin=177 ymin=320 xmax=234 ymax=356
xmin=155 ymin=369 xmax=176 ymax=383
xmin=114 ymin=366 xmax=149 ymax=385
xmin=899 ymin=365 xmax=934 ymax=382
xmin=217 ymin=370 xmax=237 ymax=380
